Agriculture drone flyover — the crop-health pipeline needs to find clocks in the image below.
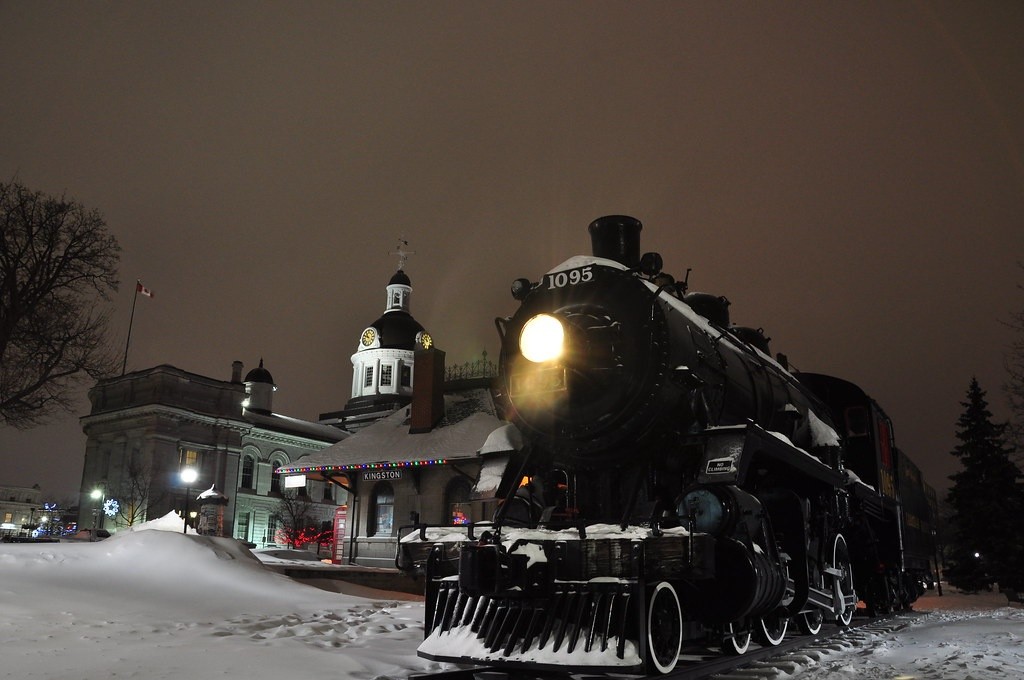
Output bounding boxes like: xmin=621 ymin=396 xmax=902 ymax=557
xmin=362 ymin=329 xmax=376 ymax=347
xmin=420 ymin=333 xmax=432 ymax=350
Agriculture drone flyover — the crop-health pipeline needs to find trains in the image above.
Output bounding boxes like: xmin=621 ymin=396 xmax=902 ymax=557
xmin=397 ymin=202 xmax=917 ymax=674
